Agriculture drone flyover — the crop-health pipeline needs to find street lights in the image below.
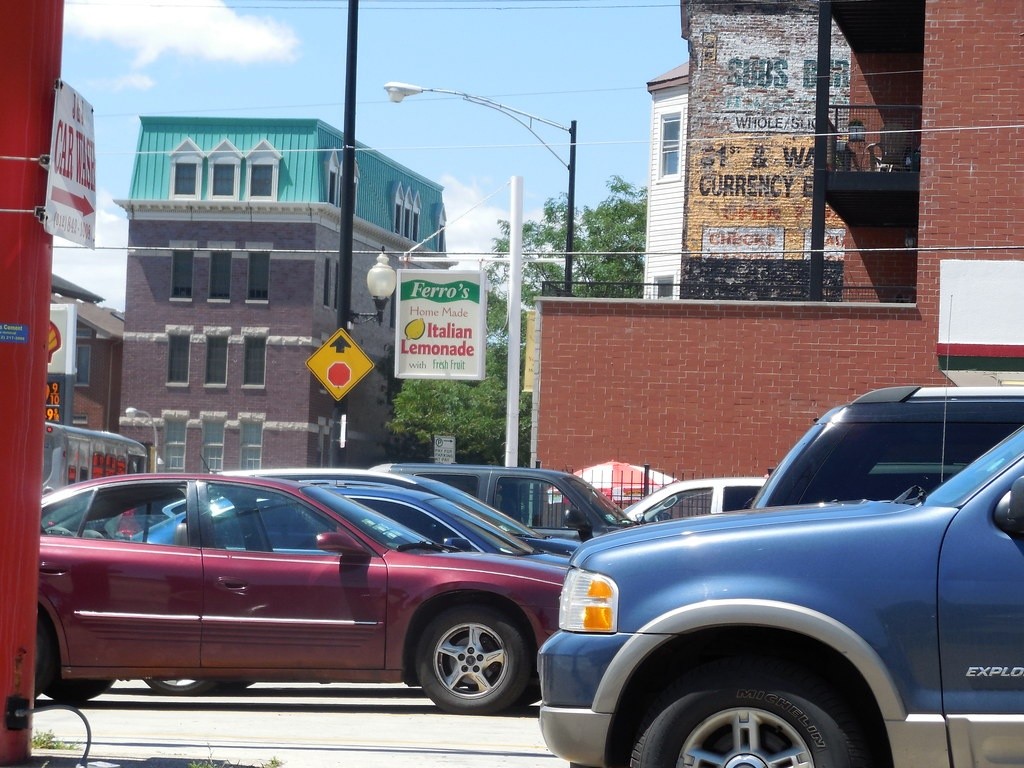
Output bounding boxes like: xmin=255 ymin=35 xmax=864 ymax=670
xmin=329 ymin=253 xmax=395 ymax=460
xmin=125 ymin=407 xmax=158 ymax=473
xmin=383 ymin=78 xmax=579 ymax=297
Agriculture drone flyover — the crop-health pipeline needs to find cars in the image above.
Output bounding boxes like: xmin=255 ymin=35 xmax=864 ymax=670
xmin=126 ymin=478 xmax=571 ymax=572
xmin=31 ymin=472 xmax=572 ymax=716
xmin=621 ymin=475 xmax=770 ymax=527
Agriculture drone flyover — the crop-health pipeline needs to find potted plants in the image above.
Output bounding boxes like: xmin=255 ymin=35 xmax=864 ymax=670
xmin=847 ymin=118 xmax=866 ymax=140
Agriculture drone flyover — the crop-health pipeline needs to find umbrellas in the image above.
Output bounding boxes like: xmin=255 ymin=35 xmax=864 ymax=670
xmin=546 ymin=459 xmax=680 ymax=505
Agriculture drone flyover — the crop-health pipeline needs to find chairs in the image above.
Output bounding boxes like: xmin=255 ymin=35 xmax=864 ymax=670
xmin=175 ymin=516 xmax=218 ymax=550
xmin=866 ymin=123 xmax=911 ymax=172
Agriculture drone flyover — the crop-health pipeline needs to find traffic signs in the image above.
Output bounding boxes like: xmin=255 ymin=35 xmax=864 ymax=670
xmin=42 ymin=77 xmax=97 ymax=253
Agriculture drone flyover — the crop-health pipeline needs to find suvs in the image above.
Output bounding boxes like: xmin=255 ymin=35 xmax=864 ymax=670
xmin=534 ymin=423 xmax=1024 ymax=768
xmin=751 ymin=385 xmax=1024 ymax=510
xmin=367 ymin=462 xmax=640 ymax=545
xmin=113 ymin=467 xmax=583 ymax=559
